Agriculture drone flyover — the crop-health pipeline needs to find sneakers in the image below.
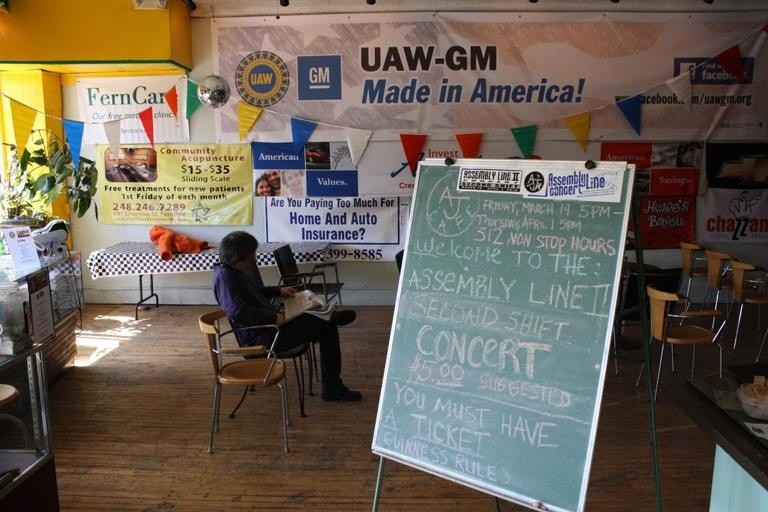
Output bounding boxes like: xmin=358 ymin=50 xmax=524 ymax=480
xmin=322 ymin=385 xmax=362 ymax=401
xmin=336 ymin=310 xmax=356 ymax=326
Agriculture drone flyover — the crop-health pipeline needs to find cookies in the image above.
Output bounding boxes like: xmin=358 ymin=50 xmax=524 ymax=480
xmin=743 ymin=375 xmax=768 ymax=399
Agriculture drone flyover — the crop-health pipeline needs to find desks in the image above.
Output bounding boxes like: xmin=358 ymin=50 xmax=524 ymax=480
xmin=87 ymin=242 xmax=331 ymax=321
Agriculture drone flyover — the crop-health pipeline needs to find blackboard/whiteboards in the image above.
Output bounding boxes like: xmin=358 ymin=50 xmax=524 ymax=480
xmin=370 ymin=157 xmax=635 ymax=512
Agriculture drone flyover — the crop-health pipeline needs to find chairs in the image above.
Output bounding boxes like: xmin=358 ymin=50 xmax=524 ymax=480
xmin=610 ymin=242 xmax=768 ymax=403
xmin=199 ymin=244 xmax=344 ymax=454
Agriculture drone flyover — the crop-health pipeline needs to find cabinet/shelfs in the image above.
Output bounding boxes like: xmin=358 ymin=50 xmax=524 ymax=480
xmin=0 ymin=251 xmax=85 ymax=512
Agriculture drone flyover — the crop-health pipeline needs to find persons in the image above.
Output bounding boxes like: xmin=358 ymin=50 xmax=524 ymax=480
xmin=213 ymin=231 xmax=362 ymax=401
xmin=256 ymin=170 xmax=306 ymax=194
